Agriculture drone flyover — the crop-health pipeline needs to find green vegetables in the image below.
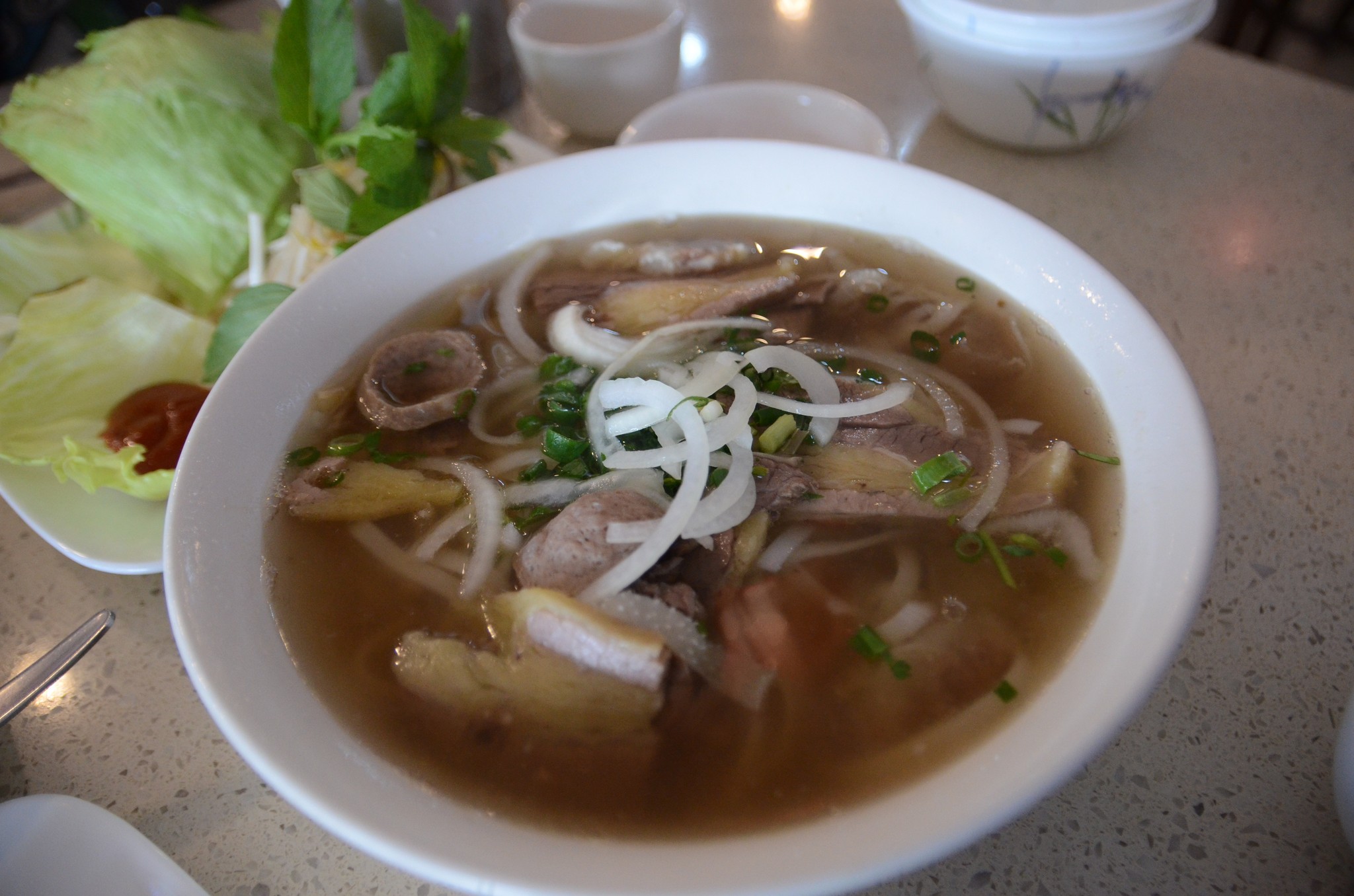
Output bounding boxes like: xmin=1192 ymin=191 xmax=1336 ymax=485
xmin=202 ymin=0 xmax=517 ymax=381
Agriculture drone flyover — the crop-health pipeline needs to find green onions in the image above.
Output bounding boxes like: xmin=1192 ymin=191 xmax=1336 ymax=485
xmin=278 ymin=273 xmax=1124 ymax=703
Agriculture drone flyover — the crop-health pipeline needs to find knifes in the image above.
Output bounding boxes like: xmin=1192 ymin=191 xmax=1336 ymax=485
xmin=0 ymin=608 xmax=116 ymax=724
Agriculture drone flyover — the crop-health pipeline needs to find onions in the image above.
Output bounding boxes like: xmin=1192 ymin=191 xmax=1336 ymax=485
xmin=349 ymin=245 xmax=1044 ymax=665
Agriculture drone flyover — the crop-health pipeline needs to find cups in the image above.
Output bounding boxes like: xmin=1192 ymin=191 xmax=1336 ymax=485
xmin=618 ymin=79 xmax=894 ymax=156
xmin=349 ymin=0 xmax=523 ymax=116
xmin=506 ymin=0 xmax=684 ymax=138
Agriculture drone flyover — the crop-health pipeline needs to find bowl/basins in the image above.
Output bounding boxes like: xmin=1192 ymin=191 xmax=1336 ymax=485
xmin=162 ymin=138 xmax=1219 ymax=896
xmin=900 ymin=2 xmax=1220 ymax=154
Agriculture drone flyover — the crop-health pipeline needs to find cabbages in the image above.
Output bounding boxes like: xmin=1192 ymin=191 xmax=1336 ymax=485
xmin=0 ymin=0 xmax=299 ymax=507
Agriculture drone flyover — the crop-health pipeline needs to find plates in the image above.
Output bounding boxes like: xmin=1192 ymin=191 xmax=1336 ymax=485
xmin=0 ymin=113 xmax=564 ymax=579
xmin=0 ymin=795 xmax=207 ymax=896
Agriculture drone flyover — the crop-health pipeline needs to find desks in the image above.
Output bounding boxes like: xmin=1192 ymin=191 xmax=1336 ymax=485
xmin=0 ymin=0 xmax=1353 ymax=896
